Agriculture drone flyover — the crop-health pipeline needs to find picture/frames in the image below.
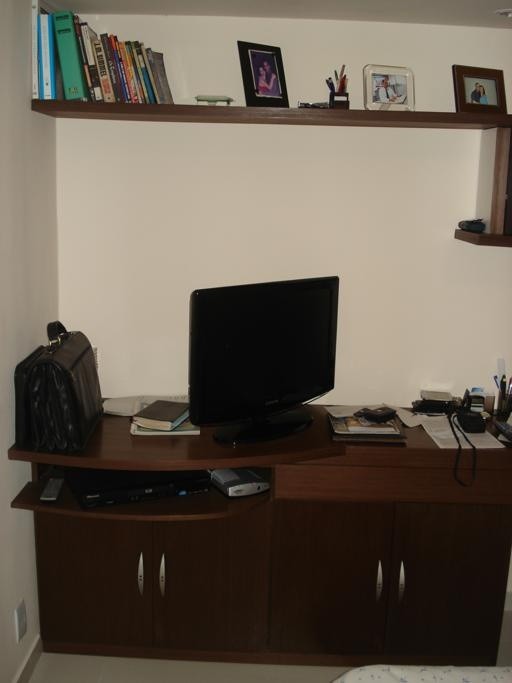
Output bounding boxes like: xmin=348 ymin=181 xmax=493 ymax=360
xmin=452 ymin=64 xmax=508 ymax=115
xmin=364 ymin=64 xmax=415 ymax=112
xmin=238 ymin=41 xmax=289 ymax=107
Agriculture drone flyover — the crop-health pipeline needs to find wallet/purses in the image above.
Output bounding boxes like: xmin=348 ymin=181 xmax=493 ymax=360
xmin=365 ymin=405 xmax=396 ymax=423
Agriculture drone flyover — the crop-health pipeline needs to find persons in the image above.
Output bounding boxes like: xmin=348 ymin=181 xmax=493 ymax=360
xmin=471 ymin=82 xmax=480 ymax=104
xmin=257 ymin=66 xmax=277 ymax=96
xmin=378 ymin=79 xmax=398 ymax=103
xmin=479 ymin=85 xmax=488 ymax=105
xmin=259 ymin=61 xmax=280 ymax=97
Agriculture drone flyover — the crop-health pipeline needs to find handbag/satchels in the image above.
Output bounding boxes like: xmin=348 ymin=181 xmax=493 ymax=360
xmin=14 ymin=344 xmax=46 ymax=449
xmin=28 ymin=321 xmax=104 ymax=452
xmin=457 ymin=411 xmax=485 ymax=433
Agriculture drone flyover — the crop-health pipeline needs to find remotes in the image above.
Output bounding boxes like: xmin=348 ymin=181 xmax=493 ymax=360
xmin=39 ymin=474 xmax=61 ymax=505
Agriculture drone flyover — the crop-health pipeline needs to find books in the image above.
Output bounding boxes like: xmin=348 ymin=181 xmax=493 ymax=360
xmin=328 ymin=413 xmax=400 ymax=435
xmin=37 ymin=9 xmax=174 ymax=105
xmin=129 ymin=418 xmax=201 ymax=436
xmin=345 ymin=416 xmax=396 ymax=432
xmin=131 ymin=400 xmax=190 ymax=431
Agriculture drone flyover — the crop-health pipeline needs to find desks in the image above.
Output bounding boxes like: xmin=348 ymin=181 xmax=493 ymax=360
xmin=8 ymin=400 xmax=511 ymax=666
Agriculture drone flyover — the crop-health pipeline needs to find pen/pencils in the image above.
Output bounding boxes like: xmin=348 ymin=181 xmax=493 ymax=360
xmin=326 ymin=65 xmax=348 ymax=95
xmin=494 ymin=375 xmax=512 ymax=412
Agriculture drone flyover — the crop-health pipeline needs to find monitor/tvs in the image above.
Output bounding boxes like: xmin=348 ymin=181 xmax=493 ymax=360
xmin=187 ymin=275 xmax=341 ymax=450
